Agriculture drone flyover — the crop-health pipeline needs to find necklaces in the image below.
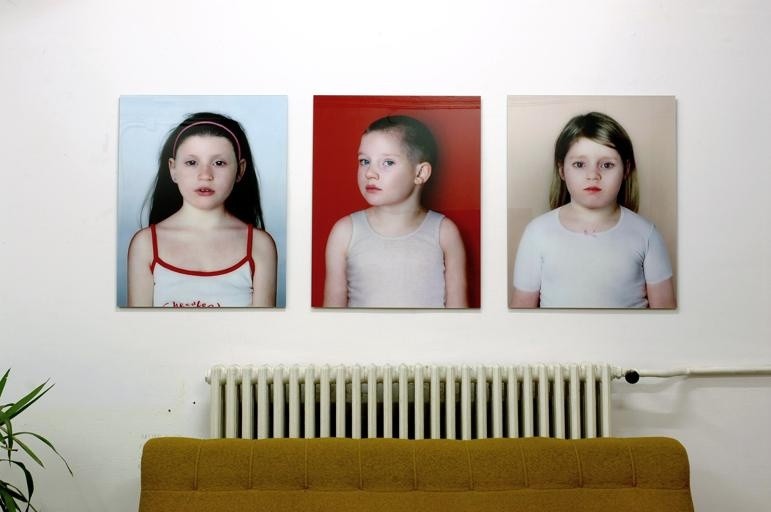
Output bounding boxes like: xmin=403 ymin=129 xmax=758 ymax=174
xmin=573 ymin=212 xmax=611 ymax=235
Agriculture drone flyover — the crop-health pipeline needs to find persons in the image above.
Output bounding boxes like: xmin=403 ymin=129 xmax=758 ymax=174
xmin=128 ymin=112 xmax=277 ymax=307
xmin=323 ymin=114 xmax=468 ymax=309
xmin=513 ymin=110 xmax=676 ymax=308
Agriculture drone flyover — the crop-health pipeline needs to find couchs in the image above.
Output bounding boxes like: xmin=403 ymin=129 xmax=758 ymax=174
xmin=137 ymin=435 xmax=696 ymax=511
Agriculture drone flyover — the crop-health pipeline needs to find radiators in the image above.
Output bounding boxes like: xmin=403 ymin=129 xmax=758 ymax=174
xmin=206 ymin=360 xmax=614 ymax=439
